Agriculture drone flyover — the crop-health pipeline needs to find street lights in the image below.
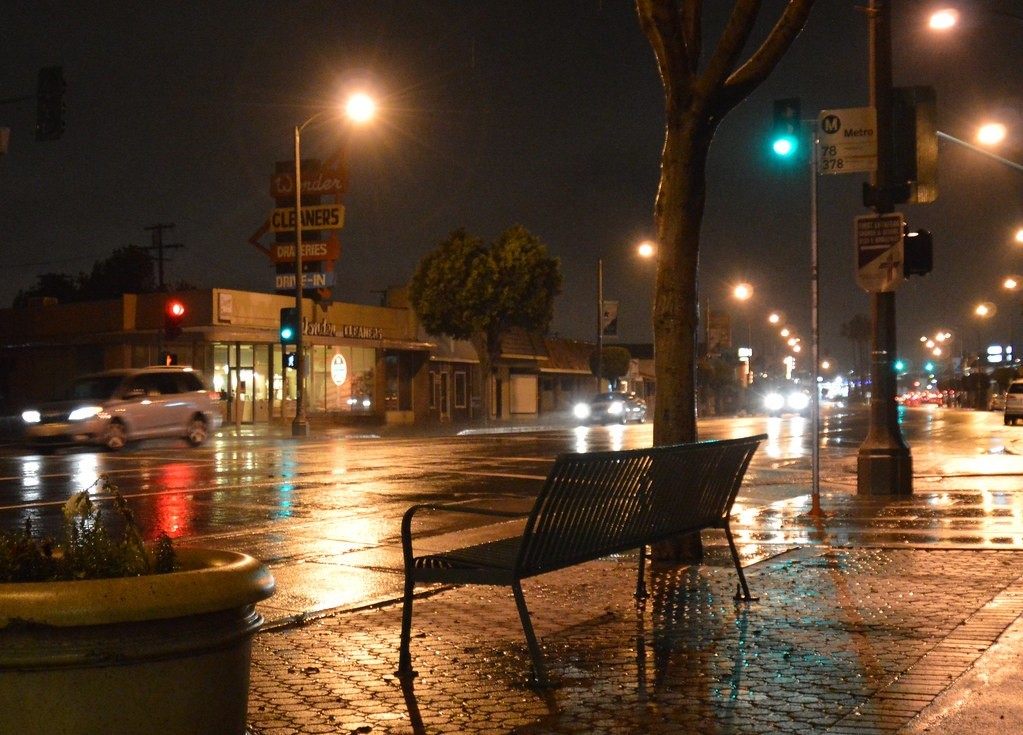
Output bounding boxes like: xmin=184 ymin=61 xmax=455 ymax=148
xmin=705 ymin=284 xmax=746 ymax=366
xmin=596 ymin=244 xmax=653 ymax=393
xmin=290 ymin=89 xmax=376 ymax=438
xmin=747 ymin=313 xmax=779 ymax=373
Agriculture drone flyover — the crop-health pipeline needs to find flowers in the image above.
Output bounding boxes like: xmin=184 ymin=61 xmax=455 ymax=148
xmin=0 ymin=469 xmax=184 ymax=585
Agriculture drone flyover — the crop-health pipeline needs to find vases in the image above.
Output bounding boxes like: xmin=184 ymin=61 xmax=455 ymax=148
xmin=0 ymin=552 xmax=278 ymax=735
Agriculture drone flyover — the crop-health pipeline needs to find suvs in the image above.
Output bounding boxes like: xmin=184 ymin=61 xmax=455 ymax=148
xmin=15 ymin=366 xmax=224 ymax=452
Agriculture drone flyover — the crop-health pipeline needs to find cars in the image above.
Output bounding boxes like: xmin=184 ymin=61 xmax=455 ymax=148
xmin=767 ymin=383 xmax=812 ymax=418
xmin=577 ymin=391 xmax=648 ymax=426
xmin=988 ymin=393 xmax=1006 ymax=412
xmin=1003 ymin=379 xmax=1023 ymax=426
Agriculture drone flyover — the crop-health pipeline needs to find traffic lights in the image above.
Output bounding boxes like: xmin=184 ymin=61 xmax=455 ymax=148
xmin=280 ymin=307 xmax=300 ymax=344
xmin=283 ymin=353 xmax=296 ymax=369
xmin=169 ymin=298 xmax=188 ymax=340
xmin=771 ymin=97 xmax=804 ymax=168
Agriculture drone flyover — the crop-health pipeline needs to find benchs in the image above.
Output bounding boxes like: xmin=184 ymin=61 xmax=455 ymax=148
xmin=399 ymin=435 xmax=764 ymax=680
